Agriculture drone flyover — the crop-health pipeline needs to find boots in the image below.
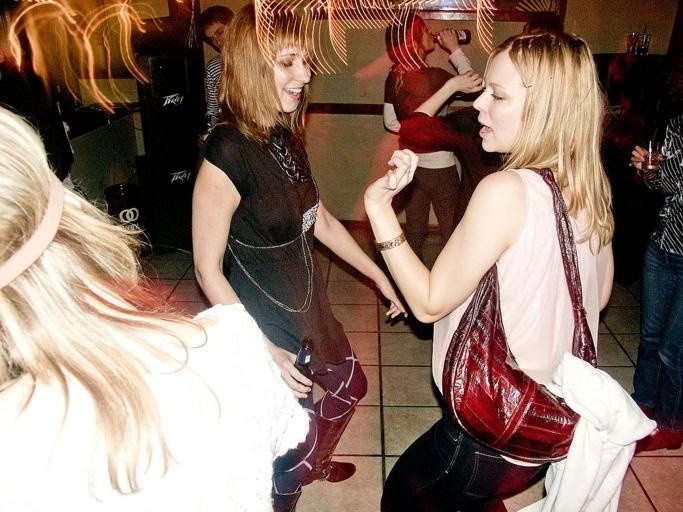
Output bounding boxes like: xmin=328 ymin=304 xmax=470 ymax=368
xmin=271 ymin=492 xmax=303 ymax=512
xmin=300 ymin=410 xmax=356 ymax=485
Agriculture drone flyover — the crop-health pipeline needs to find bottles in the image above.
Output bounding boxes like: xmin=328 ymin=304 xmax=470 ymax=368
xmin=431 ymin=29 xmax=471 ymax=44
xmin=292 ymin=339 xmax=312 ymax=407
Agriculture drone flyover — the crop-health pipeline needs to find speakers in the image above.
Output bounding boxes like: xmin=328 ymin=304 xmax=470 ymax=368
xmin=134 ymin=48 xmax=207 ymax=251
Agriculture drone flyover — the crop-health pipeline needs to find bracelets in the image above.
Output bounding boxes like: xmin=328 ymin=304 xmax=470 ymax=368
xmin=372 ymin=230 xmax=408 ymax=252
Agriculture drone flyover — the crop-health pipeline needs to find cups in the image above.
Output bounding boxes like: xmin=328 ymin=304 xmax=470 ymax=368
xmin=636 ymin=34 xmax=651 ymax=56
xmin=626 ymin=32 xmax=638 ymax=55
xmin=640 ymin=142 xmax=661 ymax=172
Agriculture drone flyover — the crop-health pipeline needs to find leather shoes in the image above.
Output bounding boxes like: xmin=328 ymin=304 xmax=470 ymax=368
xmin=636 ymin=403 xmax=681 ymax=452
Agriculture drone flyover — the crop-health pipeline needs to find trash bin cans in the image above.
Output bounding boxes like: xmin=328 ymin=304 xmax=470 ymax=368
xmin=105 ymin=183 xmax=151 ymax=258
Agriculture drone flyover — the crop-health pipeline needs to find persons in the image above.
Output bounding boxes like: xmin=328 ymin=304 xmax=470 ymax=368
xmin=362 ymin=24 xmax=617 ymax=512
xmin=520 ymin=11 xmax=566 ymax=32
xmin=187 ymin=1 xmax=411 ymax=512
xmin=383 ymin=12 xmax=476 ymax=266
xmin=626 ymin=113 xmax=683 ymax=455
xmin=191 ymin=4 xmax=236 ymax=133
xmin=0 ymin=102 xmax=314 ymax=512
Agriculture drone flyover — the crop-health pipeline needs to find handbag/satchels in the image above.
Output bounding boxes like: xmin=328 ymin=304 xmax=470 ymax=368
xmin=444 ymin=276 xmax=597 ymax=464
